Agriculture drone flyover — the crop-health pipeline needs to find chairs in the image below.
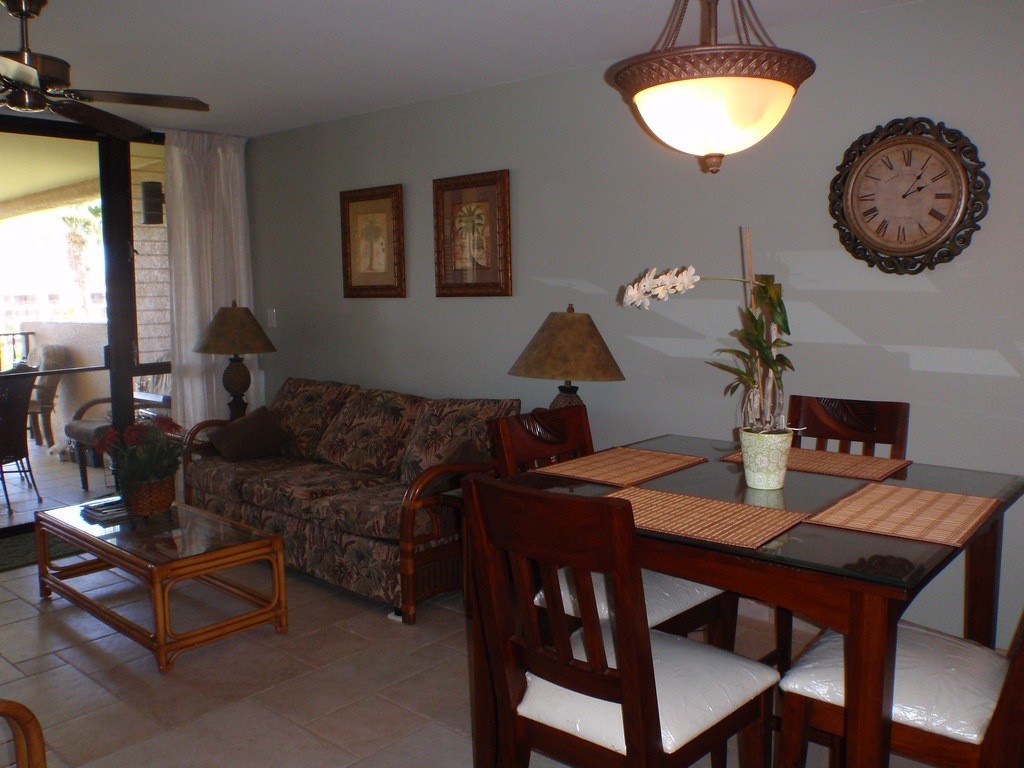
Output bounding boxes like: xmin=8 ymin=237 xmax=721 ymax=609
xmin=0 ymin=365 xmax=44 ymax=514
xmin=20 ymin=345 xmax=67 ymax=449
xmin=463 ymin=473 xmax=780 ymax=768
xmin=775 ymin=395 xmax=910 ymax=672
xmin=65 ymin=396 xmax=172 ymax=490
xmin=487 ymin=404 xmax=739 ymax=654
xmin=773 ymin=610 xmax=1024 ymax=768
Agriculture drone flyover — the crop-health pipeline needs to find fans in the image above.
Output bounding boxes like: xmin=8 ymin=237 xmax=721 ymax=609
xmin=0 ymin=0 xmax=209 ymax=143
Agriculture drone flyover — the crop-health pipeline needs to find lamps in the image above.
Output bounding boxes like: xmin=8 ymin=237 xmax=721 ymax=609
xmin=605 ymin=1 xmax=815 ymax=173
xmin=190 ymin=301 xmax=279 ymax=419
xmin=509 ymin=303 xmax=628 ymax=410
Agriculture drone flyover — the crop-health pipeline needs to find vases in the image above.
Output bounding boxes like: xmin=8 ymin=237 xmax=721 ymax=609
xmin=122 ymin=478 xmax=177 ymax=517
xmin=738 ymin=429 xmax=793 ymax=489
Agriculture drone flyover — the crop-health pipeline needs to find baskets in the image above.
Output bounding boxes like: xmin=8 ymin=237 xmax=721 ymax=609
xmin=117 ymin=475 xmax=175 ymax=515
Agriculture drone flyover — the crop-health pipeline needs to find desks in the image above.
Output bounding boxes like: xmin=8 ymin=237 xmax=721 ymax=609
xmin=441 ymin=435 xmax=1024 ymax=768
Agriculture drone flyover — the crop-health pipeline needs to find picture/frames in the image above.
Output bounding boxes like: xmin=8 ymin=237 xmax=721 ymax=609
xmin=339 ymin=184 xmax=407 ymax=299
xmin=433 ymin=166 xmax=511 ymax=297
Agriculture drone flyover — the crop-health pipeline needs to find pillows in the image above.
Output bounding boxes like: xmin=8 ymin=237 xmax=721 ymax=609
xmin=205 ymin=406 xmax=290 ymax=463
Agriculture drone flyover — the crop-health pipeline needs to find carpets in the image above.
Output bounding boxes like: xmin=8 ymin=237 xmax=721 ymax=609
xmin=0 ymin=523 xmax=87 ymax=573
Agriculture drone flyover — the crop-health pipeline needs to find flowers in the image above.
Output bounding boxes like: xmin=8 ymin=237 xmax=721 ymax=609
xmin=93 ymin=415 xmax=183 ymax=483
xmin=622 ymin=265 xmax=793 ymax=429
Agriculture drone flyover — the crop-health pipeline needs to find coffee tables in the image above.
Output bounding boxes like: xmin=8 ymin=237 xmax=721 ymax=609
xmin=34 ymin=493 xmax=289 ymax=674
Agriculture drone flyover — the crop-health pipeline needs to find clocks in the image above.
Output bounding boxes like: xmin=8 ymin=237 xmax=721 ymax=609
xmin=829 ymin=116 xmax=990 ymax=277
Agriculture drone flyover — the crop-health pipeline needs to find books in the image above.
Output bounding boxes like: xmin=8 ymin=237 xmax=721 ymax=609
xmin=80 ymin=495 xmax=129 ymax=521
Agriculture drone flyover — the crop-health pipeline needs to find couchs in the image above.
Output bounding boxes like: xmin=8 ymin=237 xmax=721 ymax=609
xmin=184 ymin=377 xmax=520 ymax=624
